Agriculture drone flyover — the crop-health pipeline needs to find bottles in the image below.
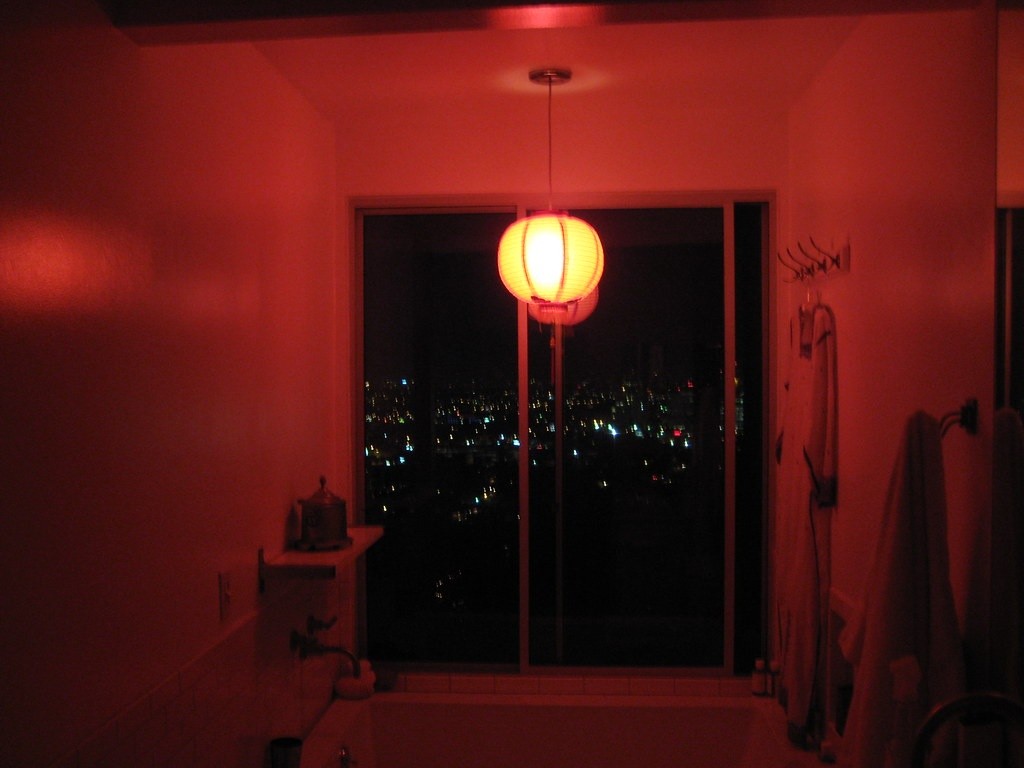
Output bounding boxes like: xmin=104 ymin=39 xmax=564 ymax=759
xmin=766 ymin=662 xmax=780 ymax=695
xmin=750 ymin=660 xmax=767 ymax=695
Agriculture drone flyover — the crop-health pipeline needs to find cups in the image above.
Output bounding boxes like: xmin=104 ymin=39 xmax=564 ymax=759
xmin=270 ymin=737 xmax=303 ymax=768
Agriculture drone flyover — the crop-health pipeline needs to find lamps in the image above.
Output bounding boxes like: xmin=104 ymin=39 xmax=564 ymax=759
xmin=497 ymin=68 xmax=605 ymax=311
